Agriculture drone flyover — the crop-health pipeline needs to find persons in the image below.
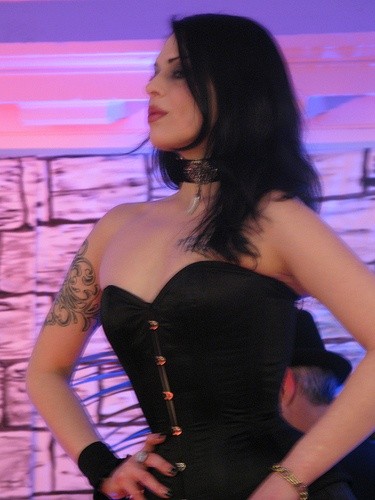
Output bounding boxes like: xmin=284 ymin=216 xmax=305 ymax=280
xmin=26 ymin=11 xmax=375 ymax=500
xmin=277 ymin=307 xmax=375 ymax=500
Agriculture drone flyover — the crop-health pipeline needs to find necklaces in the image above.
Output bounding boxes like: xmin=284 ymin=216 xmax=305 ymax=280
xmin=180 ymin=158 xmax=221 ymax=216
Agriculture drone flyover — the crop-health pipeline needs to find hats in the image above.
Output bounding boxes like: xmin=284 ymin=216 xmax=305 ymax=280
xmin=286 ymin=310 xmax=352 ymax=386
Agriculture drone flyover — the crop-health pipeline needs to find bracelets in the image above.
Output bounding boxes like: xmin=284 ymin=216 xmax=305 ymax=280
xmin=77 ymin=441 xmax=130 ymax=489
xmin=270 ymin=464 xmax=308 ymax=500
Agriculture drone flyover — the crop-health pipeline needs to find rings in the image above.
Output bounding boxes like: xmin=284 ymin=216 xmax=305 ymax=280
xmin=133 ymin=451 xmax=149 ymax=465
xmin=128 ymin=489 xmax=145 ymax=499
